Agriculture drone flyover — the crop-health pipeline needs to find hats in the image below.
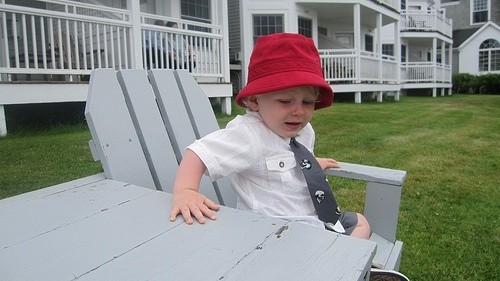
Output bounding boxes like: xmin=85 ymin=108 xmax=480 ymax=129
xmin=235 ymin=33 xmax=334 ymax=110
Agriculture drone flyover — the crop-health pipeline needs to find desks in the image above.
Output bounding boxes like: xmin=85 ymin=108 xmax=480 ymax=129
xmin=0 ymin=173 xmax=378 ymax=281
xmin=10 ymin=52 xmax=65 ymax=82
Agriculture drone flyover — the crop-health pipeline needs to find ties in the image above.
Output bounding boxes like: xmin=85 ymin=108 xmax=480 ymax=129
xmin=289 ymin=137 xmax=341 ymax=225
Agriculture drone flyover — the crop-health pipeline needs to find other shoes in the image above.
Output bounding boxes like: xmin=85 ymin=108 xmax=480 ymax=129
xmin=363 ymin=265 xmax=410 ymax=281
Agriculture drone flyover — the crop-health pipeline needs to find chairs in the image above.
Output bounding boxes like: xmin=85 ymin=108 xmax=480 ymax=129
xmin=85 ymin=67 xmax=407 ymax=272
xmin=326 ymin=62 xmax=354 ymax=84
xmin=70 ymin=36 xmax=104 ymax=78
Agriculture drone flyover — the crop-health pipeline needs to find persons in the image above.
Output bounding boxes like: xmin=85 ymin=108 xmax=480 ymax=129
xmin=144 ymin=20 xmax=196 ymax=72
xmin=170 ymin=32 xmax=409 ymax=281
